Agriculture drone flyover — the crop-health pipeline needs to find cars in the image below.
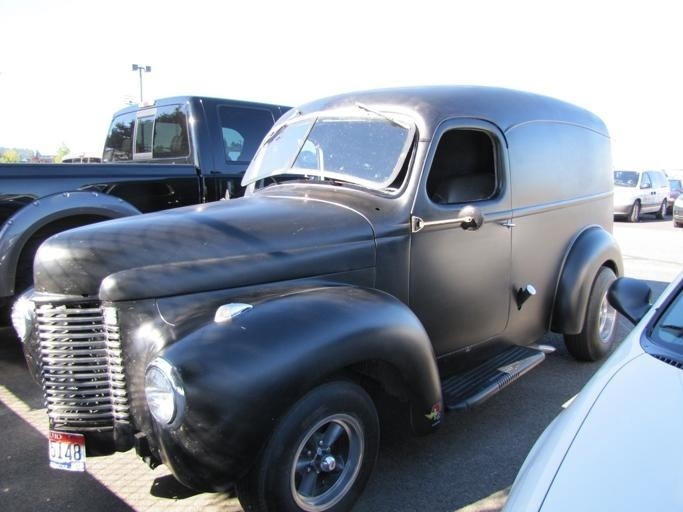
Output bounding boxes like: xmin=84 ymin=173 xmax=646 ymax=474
xmin=8 ymin=88 xmax=625 ymax=512
xmin=612 ymin=168 xmax=682 ymax=228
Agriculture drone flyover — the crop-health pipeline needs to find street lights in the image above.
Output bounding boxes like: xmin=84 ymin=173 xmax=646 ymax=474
xmin=128 ymin=62 xmax=148 ymax=99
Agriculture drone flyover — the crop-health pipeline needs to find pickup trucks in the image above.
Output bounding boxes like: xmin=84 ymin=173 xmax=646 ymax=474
xmin=1 ymin=93 xmax=315 ymax=349
